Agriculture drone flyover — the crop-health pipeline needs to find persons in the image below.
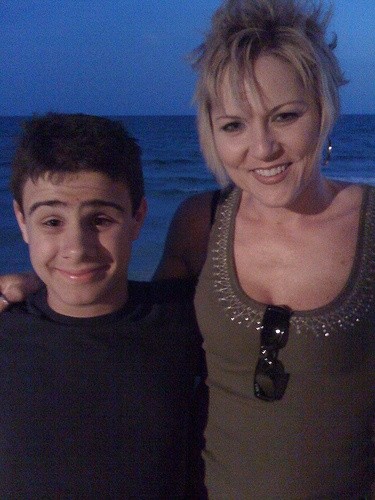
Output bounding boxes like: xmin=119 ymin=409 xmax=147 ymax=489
xmin=0 ymin=0 xmax=375 ymax=500
xmin=0 ymin=115 xmax=208 ymax=500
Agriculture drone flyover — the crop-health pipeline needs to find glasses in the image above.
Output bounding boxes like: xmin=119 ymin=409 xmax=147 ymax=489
xmin=254 ymin=305 xmax=290 ymax=402
